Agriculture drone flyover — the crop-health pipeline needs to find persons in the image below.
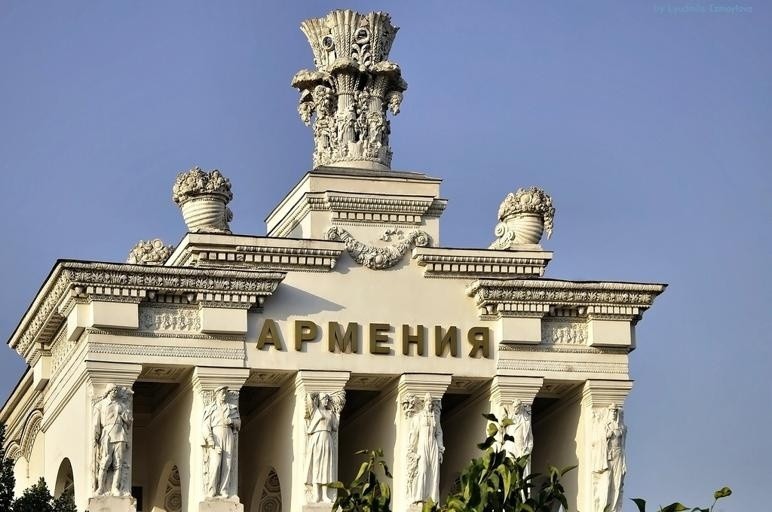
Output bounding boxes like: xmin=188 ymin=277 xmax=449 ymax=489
xmin=303 ymin=392 xmax=338 ymax=504
xmin=592 ymin=402 xmax=627 ymax=512
xmin=93 ymin=382 xmax=132 ymax=497
xmin=500 ymin=398 xmax=534 ymax=504
xmin=202 ymin=385 xmax=241 ymax=498
xmin=407 ymin=392 xmax=446 ymax=508
